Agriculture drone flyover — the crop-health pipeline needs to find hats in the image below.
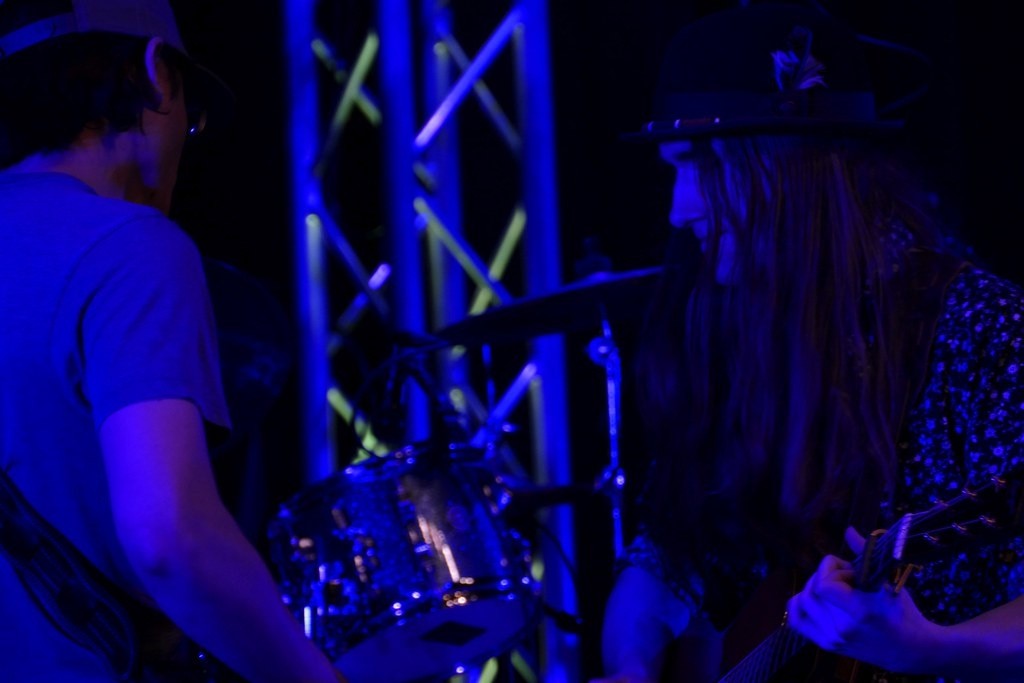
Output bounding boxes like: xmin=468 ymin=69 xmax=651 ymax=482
xmin=617 ymin=6 xmax=905 ymax=144
xmin=0 ymin=0 xmax=222 ymax=88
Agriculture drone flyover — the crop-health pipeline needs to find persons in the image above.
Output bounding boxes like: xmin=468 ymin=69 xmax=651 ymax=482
xmin=602 ymin=0 xmax=1024 ymax=683
xmin=0 ymin=0 xmax=344 ymax=683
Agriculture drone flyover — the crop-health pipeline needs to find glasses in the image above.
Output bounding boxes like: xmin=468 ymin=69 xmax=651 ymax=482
xmin=129 ymin=38 xmax=210 ymax=137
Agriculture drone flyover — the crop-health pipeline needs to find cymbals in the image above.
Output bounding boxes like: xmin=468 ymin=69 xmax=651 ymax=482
xmin=199 ymin=256 xmax=295 ymax=458
xmin=434 ymin=262 xmax=670 ymax=349
xmin=352 ymin=324 xmax=484 ymax=389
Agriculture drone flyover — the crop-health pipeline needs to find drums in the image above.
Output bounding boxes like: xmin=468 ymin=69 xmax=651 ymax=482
xmin=271 ymin=437 xmax=543 ymax=682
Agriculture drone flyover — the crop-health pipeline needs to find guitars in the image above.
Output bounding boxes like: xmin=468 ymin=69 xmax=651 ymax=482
xmin=715 ymin=458 xmax=1024 ymax=682
